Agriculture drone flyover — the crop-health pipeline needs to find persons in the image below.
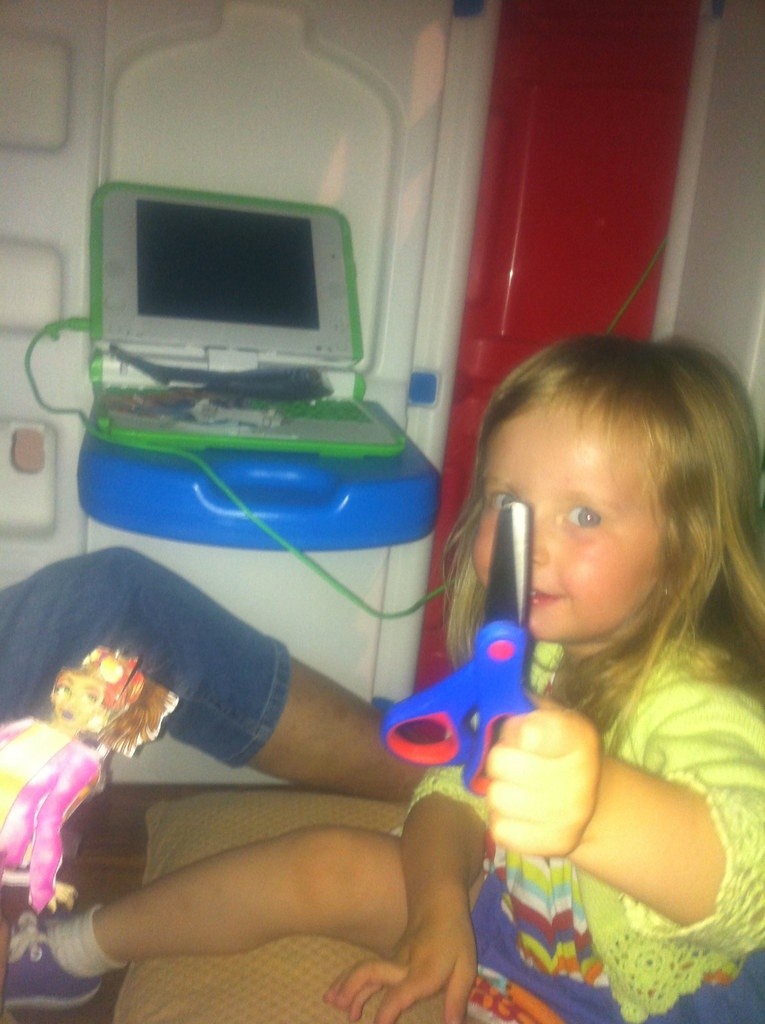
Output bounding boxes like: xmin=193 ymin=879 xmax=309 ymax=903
xmin=1 ymin=332 xmax=765 ymax=1024
xmin=1 ymin=545 xmax=432 ymax=801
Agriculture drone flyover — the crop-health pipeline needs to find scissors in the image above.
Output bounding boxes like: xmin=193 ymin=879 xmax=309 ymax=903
xmin=376 ymin=498 xmax=545 ymax=800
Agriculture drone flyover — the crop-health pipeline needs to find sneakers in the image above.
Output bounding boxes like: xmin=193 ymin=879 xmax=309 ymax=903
xmin=2 ymin=915 xmax=101 ymax=1009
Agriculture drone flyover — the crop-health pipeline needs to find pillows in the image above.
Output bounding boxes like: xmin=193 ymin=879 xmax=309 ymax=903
xmin=111 ymin=791 xmax=481 ymax=1024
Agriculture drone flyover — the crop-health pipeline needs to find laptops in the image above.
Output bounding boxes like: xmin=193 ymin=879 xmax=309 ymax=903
xmin=89 ymin=182 xmax=407 ymax=457
